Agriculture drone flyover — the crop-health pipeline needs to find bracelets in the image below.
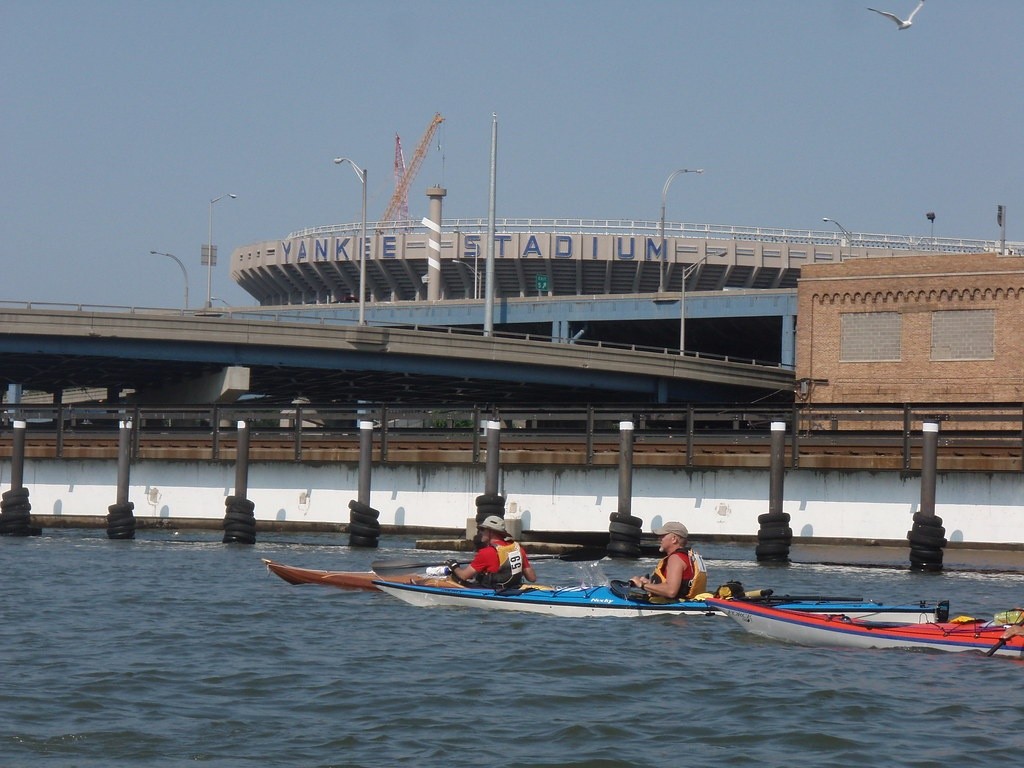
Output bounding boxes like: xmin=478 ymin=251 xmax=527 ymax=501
xmin=641 ymin=583 xmax=648 ymax=590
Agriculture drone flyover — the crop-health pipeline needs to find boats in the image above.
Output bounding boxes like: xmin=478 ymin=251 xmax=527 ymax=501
xmin=368 ymin=576 xmax=731 ymax=620
xmin=703 ymin=589 xmax=1024 ymax=659
xmin=258 ymin=554 xmax=563 ymax=593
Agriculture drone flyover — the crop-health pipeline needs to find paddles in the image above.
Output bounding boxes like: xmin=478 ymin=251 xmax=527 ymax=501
xmin=986 ymin=619 xmax=1024 ymax=657
xmin=608 ymin=578 xmax=706 ymax=596
xmin=370 ymin=555 xmax=566 ymax=578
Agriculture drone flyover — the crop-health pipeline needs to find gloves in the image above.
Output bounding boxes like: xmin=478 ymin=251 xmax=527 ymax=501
xmin=445 ymin=558 xmax=460 ymax=572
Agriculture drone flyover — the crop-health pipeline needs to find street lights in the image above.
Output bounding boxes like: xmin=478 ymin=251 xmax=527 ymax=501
xmin=207 ymin=190 xmax=238 ymax=307
xmin=452 ymin=229 xmax=478 ymax=300
xmin=149 ymin=249 xmax=190 ymax=309
xmin=680 ymin=250 xmax=730 ymax=355
xmin=821 ymin=217 xmax=853 ymax=259
xmin=926 ymin=212 xmax=935 ymax=252
xmin=658 ymin=167 xmax=704 ymax=289
xmin=452 ymin=259 xmax=482 ymax=299
xmin=333 ymin=155 xmax=368 ymax=325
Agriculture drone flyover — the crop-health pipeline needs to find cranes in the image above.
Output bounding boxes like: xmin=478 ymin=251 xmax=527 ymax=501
xmin=392 ymin=129 xmax=411 ymax=232
xmin=372 ymin=112 xmax=446 ymax=237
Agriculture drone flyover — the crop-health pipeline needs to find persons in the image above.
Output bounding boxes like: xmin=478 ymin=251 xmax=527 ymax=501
xmin=629 ymin=521 xmax=707 ymax=603
xmin=445 ymin=516 xmax=537 ymax=588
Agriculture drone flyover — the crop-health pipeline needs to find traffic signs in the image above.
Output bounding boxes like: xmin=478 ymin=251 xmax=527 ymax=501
xmin=536 ymin=273 xmax=548 ymax=291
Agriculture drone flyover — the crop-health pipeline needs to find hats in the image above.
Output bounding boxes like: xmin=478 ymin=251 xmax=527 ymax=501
xmin=476 ymin=515 xmax=512 ymax=537
xmin=652 ymin=522 xmax=688 ymax=540
